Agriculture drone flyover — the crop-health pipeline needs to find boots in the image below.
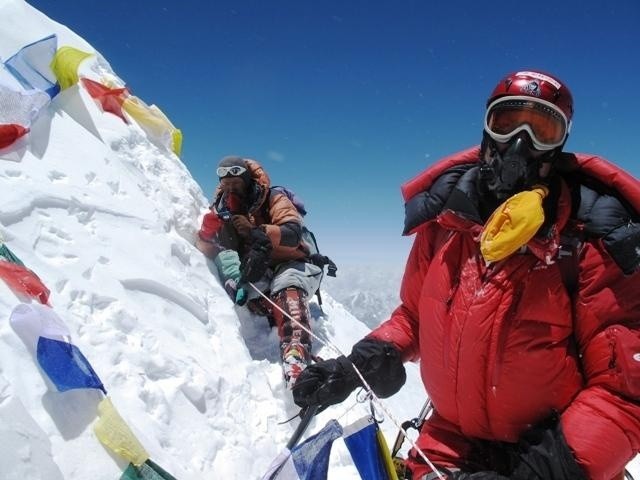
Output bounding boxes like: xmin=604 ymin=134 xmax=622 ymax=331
xmin=247 ymin=285 xmax=312 ymax=392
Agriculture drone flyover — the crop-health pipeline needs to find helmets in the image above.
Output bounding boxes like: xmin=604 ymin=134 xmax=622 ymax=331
xmin=486 ymin=70 xmax=573 ymax=123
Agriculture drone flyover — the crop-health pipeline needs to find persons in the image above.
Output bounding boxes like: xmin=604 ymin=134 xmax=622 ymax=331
xmin=288 ymin=67 xmax=640 ymax=480
xmin=193 ymin=153 xmax=328 ymax=387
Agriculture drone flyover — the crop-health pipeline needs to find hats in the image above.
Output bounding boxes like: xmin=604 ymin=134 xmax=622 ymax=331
xmin=218 ymin=156 xmax=251 ymax=190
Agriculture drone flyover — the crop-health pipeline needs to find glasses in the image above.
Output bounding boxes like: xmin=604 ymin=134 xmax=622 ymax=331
xmin=484 ymin=95 xmax=568 ymax=151
xmin=217 ymin=166 xmax=247 ymax=177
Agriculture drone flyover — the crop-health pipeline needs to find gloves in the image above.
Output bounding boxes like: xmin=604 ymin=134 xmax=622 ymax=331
xmin=293 ymin=337 xmax=406 ymax=406
xmin=200 ymin=208 xmax=223 ymax=242
xmin=467 ymin=409 xmax=588 ymax=479
xmin=236 ymin=229 xmax=273 ymax=291
xmin=232 ymin=215 xmax=253 ymax=236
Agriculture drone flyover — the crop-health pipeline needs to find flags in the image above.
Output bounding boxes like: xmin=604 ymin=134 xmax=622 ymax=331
xmin=9 ymin=299 xmax=73 ymax=341
xmin=91 ymin=395 xmax=150 ymax=471
xmin=0 ymin=242 xmax=27 ymax=269
xmin=1 ymin=260 xmax=54 ymax=306
xmin=374 ymin=420 xmax=401 ymax=480
xmin=340 ymin=414 xmax=391 ymax=480
xmin=2 ymin=30 xmax=184 ymax=159
xmin=290 ymin=418 xmax=344 ymax=480
xmin=118 ymin=457 xmax=178 ymax=480
xmin=36 ymin=334 xmax=108 ymax=396
